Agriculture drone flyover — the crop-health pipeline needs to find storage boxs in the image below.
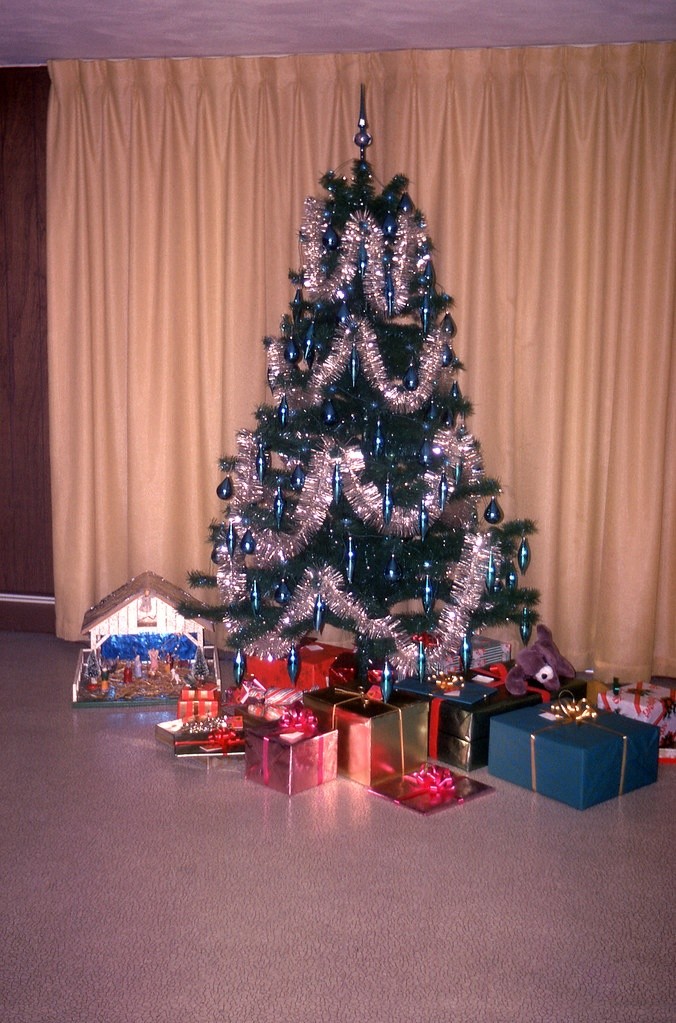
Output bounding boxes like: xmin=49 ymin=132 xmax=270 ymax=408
xmin=305 ymin=681 xmax=432 ymax=785
xmin=244 ymin=718 xmax=341 ymax=794
xmin=489 ymin=699 xmax=661 ymax=812
xmin=242 ymin=641 xmax=353 ymax=694
xmin=597 ymin=683 xmax=676 ymax=741
xmin=327 ymin=653 xmax=389 ymax=688
xmin=388 ymin=659 xmax=588 ymax=774
xmin=425 ymin=636 xmax=511 ymax=676
xmin=181 ymin=682 xmax=218 ymax=701
xmin=176 ymin=692 xmax=222 ymax=720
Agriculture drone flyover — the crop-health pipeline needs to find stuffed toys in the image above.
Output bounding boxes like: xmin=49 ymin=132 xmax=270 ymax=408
xmin=505 ymin=624 xmax=576 ymax=695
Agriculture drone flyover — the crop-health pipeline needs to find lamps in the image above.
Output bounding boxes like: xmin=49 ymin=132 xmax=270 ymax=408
xmin=139 ymin=592 xmax=153 ymax=613
